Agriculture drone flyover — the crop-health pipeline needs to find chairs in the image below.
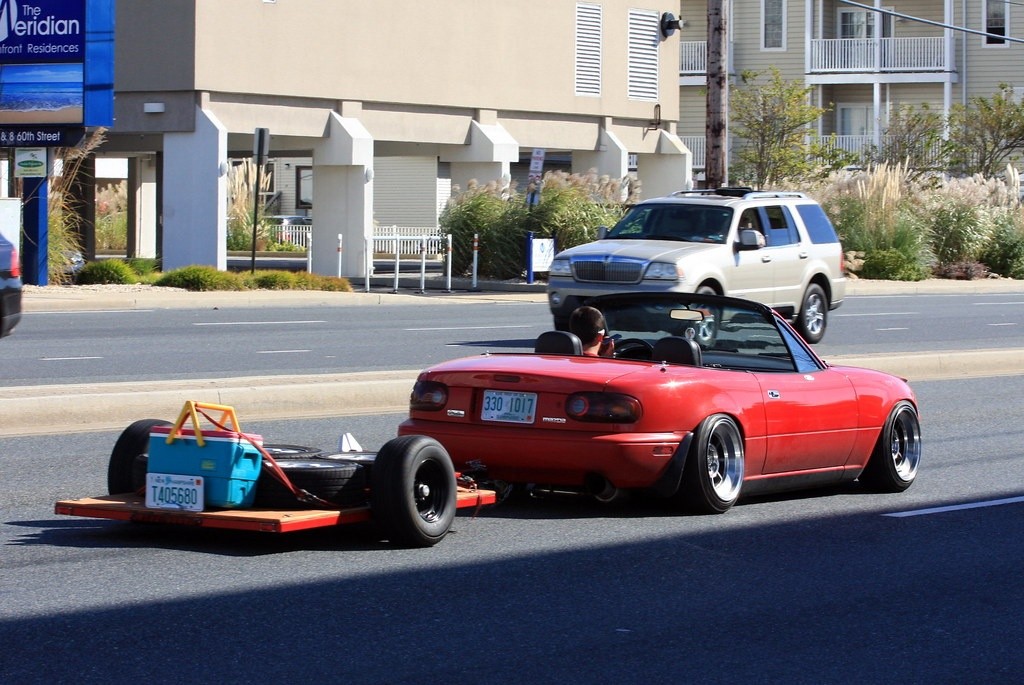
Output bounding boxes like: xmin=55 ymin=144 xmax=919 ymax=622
xmin=535 ymin=330 xmax=583 ymax=356
xmin=653 ymin=336 xmax=702 ymax=366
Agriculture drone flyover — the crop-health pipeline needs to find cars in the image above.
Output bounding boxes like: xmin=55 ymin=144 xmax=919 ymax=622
xmin=395 ymin=288 xmax=923 ymax=514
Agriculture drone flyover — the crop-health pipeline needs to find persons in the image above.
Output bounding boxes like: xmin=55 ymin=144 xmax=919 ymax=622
xmin=687 ymin=329 xmax=693 ymax=337
xmin=570 ymin=306 xmax=614 ymax=356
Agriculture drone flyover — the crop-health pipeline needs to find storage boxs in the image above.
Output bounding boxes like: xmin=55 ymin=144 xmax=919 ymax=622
xmin=147 ymin=399 xmax=263 ymax=509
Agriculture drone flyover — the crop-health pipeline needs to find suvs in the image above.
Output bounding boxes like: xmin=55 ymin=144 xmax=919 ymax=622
xmin=546 ymin=188 xmax=845 ymax=345
